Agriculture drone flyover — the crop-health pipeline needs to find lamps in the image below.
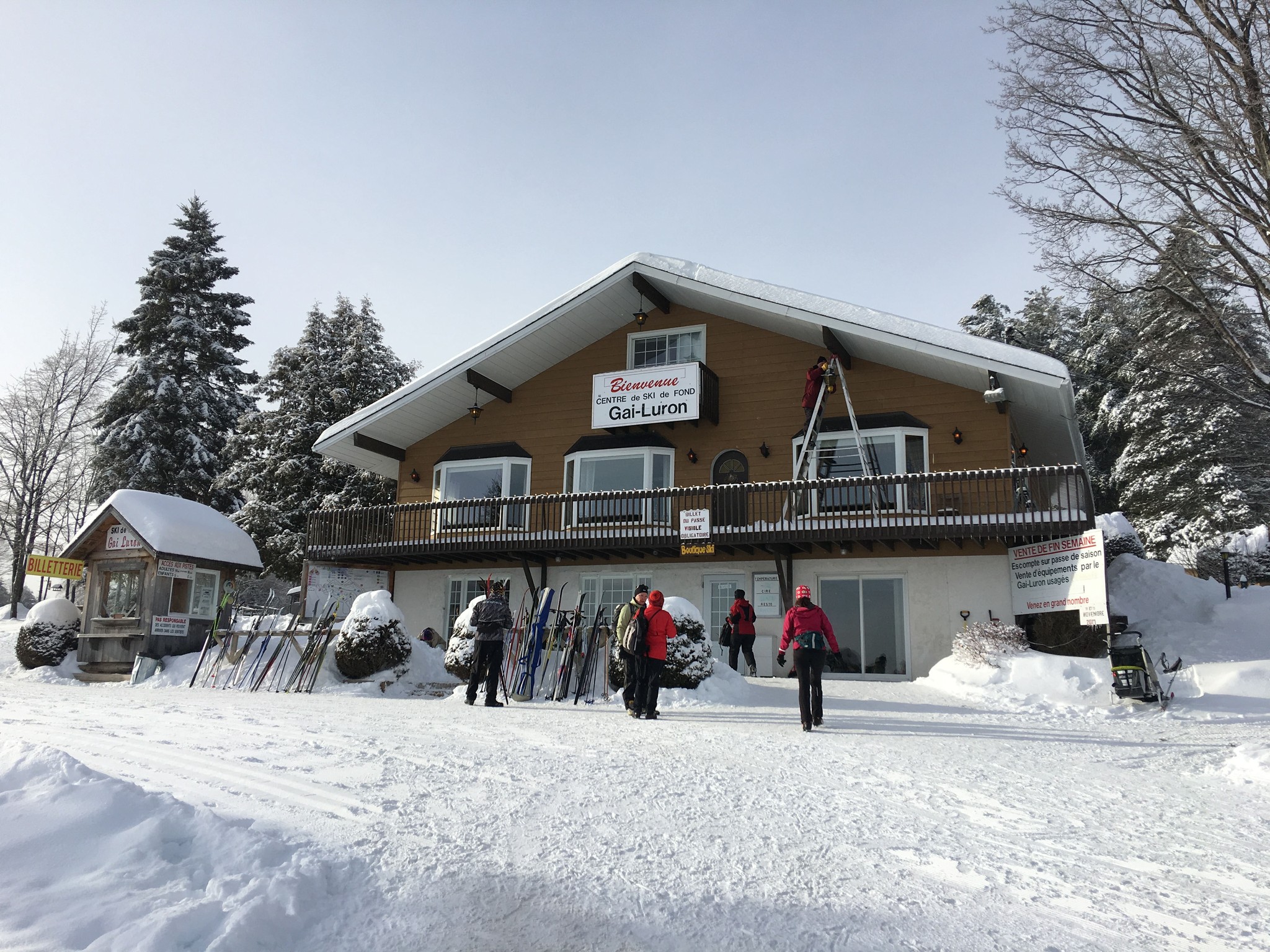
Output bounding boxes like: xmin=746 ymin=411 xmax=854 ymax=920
xmin=467 ymin=385 xmax=484 ymax=425
xmin=686 ymin=448 xmax=698 ymax=464
xmin=759 ymin=441 xmax=769 ymax=458
xmin=821 ymin=345 xmax=839 ymax=395
xmin=1018 ymin=442 xmax=1028 ymax=459
xmin=409 ymin=468 xmax=420 ymax=482
xmin=555 ymin=551 xmax=563 ymax=563
xmin=839 ymin=540 xmax=849 ymax=555
xmin=982 ymin=376 xmax=1006 ymax=404
xmin=632 ymin=290 xmax=649 ymax=331
xmin=951 ymin=426 xmax=963 ymax=444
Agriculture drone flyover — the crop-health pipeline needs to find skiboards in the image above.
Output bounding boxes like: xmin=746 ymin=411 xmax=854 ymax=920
xmin=477 ymin=572 xmax=611 ymax=705
xmin=189 ymin=586 xmax=342 ymax=695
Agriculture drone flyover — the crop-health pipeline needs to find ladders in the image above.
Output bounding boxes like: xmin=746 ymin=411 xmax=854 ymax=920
xmin=777 ymin=354 xmax=883 ymax=522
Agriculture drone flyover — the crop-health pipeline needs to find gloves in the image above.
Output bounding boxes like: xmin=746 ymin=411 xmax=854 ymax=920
xmin=821 ymin=362 xmax=828 ymax=371
xmin=619 ymin=644 xmax=629 ymax=659
xmin=834 ymin=652 xmax=845 ymax=668
xmin=777 ymin=653 xmax=787 ymax=667
xmin=827 ymin=385 xmax=833 ymax=392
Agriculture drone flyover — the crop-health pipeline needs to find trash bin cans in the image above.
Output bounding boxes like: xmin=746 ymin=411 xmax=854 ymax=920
xmin=128 ymin=651 xmax=161 ymax=684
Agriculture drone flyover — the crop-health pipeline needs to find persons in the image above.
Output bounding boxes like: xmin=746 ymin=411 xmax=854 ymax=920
xmin=725 ymin=589 xmax=757 ymax=677
xmin=464 ymin=581 xmax=513 ymax=707
xmin=417 ymin=627 xmax=446 ymax=652
xmin=802 ymin=356 xmax=836 ymax=447
xmin=616 ymin=584 xmax=660 ymax=715
xmin=775 ymin=584 xmax=843 ymax=732
xmin=630 ymin=590 xmax=677 ymax=719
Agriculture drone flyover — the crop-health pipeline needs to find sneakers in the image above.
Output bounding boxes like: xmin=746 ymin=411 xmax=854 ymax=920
xmin=464 ymin=699 xmax=474 ymax=705
xmin=802 ymin=721 xmax=812 ymax=732
xmin=803 ymin=440 xmax=816 ymax=451
xmin=632 ymin=712 xmax=641 ymax=719
xmin=485 ymin=701 xmax=503 ymax=707
xmin=813 ymin=717 xmax=824 ymax=726
xmin=645 ymin=713 xmax=657 ymax=719
xmin=750 ymin=666 xmax=757 ymax=677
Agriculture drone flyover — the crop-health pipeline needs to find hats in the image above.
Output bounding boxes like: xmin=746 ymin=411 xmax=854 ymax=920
xmin=490 ymin=581 xmax=504 ymax=595
xmin=649 ymin=590 xmax=664 ymax=606
xmin=735 ymin=589 xmax=745 ymax=598
xmin=423 ymin=630 xmax=432 ymax=640
xmin=634 ymin=584 xmax=648 ymax=597
xmin=796 ymin=585 xmax=811 ymax=600
xmin=817 ymin=356 xmax=827 ymax=365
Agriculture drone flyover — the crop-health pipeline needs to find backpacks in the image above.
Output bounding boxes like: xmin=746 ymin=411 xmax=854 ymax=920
xmin=623 ymin=607 xmax=650 ymax=660
xmin=611 ymin=602 xmax=634 ymax=638
xmin=718 ymin=623 xmax=732 ymax=656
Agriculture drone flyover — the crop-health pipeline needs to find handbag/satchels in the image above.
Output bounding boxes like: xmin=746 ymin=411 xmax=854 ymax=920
xmin=794 ymin=630 xmax=825 ymax=649
xmin=477 ymin=621 xmax=503 ymax=633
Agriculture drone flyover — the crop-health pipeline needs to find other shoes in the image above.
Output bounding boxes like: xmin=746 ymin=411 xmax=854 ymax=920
xmin=641 ymin=708 xmax=660 ymax=715
xmin=627 ymin=700 xmax=635 ymax=715
